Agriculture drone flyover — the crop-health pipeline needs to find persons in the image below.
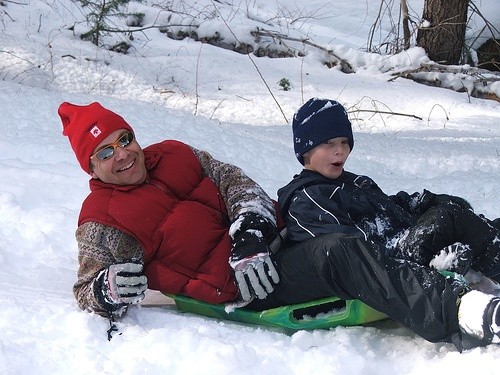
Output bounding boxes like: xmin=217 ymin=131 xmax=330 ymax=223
xmin=57 ymin=101 xmax=500 ymax=347
xmin=277 ymin=98 xmax=499 ymax=289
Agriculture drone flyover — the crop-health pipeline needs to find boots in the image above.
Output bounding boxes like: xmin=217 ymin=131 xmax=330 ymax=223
xmin=458 ymin=285 xmax=500 ymax=347
xmin=472 ymin=228 xmax=500 ymax=284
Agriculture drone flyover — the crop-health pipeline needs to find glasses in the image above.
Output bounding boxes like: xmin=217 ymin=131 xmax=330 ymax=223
xmin=88 ymin=129 xmax=136 ymax=165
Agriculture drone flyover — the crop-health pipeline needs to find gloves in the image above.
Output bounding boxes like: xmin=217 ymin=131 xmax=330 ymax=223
xmin=228 ymin=211 xmax=278 ymax=303
xmin=407 ymin=187 xmax=475 ymax=217
xmin=95 ymin=260 xmax=149 ymax=313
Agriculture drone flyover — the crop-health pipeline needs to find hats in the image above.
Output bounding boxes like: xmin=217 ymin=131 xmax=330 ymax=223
xmin=290 ymin=99 xmax=355 ymax=161
xmin=55 ymin=101 xmax=129 ymax=177
